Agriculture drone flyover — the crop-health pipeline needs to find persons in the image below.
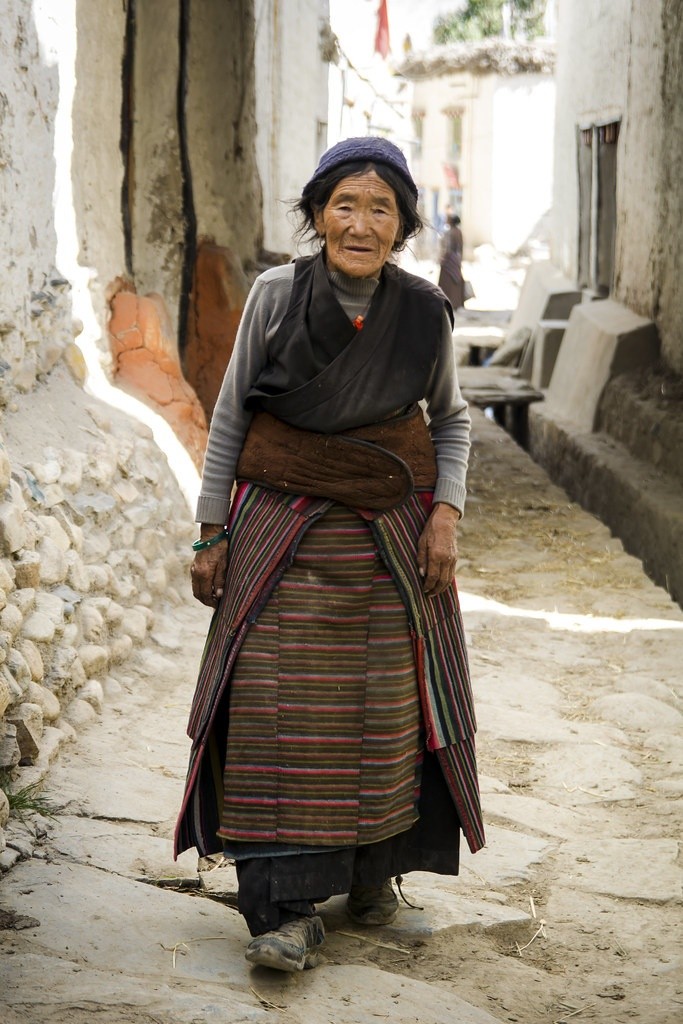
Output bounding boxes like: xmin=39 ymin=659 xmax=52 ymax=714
xmin=436 ymin=213 xmax=466 ymax=311
xmin=172 ymin=134 xmax=484 ymax=974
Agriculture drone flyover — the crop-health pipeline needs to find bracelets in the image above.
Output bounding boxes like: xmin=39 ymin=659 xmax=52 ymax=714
xmin=191 ymin=525 xmax=227 ymax=552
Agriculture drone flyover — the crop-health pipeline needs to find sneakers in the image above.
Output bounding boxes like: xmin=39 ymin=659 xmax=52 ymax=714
xmin=346 ymin=878 xmax=399 ymax=924
xmin=246 ymin=917 xmax=325 ymax=973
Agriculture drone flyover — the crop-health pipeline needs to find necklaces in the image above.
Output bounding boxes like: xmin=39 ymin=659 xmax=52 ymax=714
xmin=324 ymin=258 xmax=384 ymax=330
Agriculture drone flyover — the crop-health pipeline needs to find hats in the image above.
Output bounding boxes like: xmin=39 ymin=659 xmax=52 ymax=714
xmin=313 ymin=137 xmax=418 ymax=201
